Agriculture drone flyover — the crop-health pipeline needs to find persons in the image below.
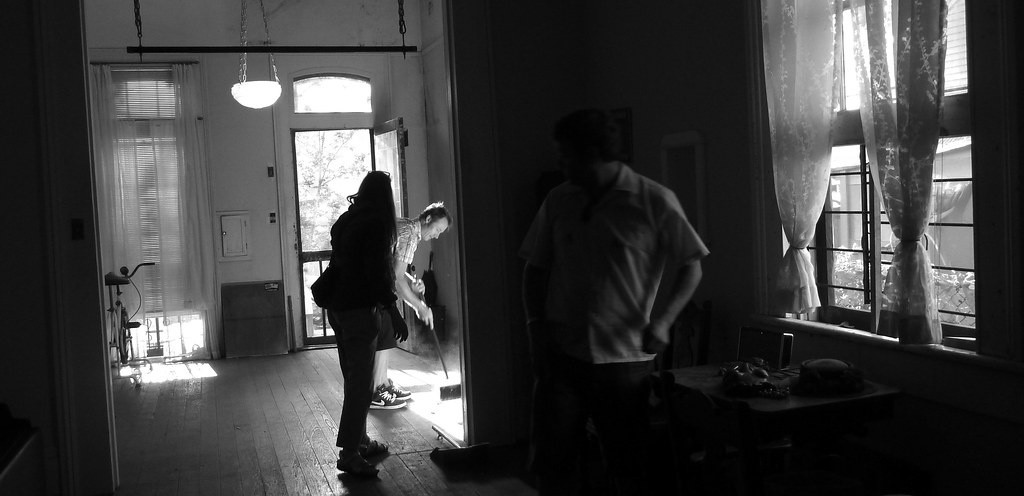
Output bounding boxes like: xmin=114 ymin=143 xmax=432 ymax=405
xmin=311 ymin=170 xmax=452 ymax=479
xmin=518 ymin=110 xmax=704 ymax=496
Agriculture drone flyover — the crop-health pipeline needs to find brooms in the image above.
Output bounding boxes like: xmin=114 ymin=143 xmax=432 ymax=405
xmin=412 ymin=273 xmax=461 ymax=401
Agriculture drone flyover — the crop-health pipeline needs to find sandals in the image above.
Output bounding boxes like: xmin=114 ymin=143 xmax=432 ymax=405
xmin=337 ymin=449 xmax=379 ymax=476
xmin=359 ymin=441 xmax=388 ymax=458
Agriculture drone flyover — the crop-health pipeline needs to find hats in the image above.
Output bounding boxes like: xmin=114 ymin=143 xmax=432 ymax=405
xmin=793 ymin=358 xmax=878 ymax=398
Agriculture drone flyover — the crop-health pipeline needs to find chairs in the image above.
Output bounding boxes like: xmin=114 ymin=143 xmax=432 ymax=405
xmin=660 ymin=380 xmax=864 ymax=496
xmin=654 ymin=301 xmax=713 ymax=496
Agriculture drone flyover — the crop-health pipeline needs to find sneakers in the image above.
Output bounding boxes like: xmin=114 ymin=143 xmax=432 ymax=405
xmin=370 ymin=378 xmax=411 ymax=409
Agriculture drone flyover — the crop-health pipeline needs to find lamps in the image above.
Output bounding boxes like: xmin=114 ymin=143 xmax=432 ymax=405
xmin=231 ymin=0 xmax=282 ymax=110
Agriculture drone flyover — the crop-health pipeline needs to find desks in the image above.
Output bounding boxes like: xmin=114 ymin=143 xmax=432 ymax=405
xmin=675 ymin=363 xmax=905 ymax=496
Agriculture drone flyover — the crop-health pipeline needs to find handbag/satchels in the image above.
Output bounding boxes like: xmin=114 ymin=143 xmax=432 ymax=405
xmin=311 ymin=267 xmax=346 ymax=310
